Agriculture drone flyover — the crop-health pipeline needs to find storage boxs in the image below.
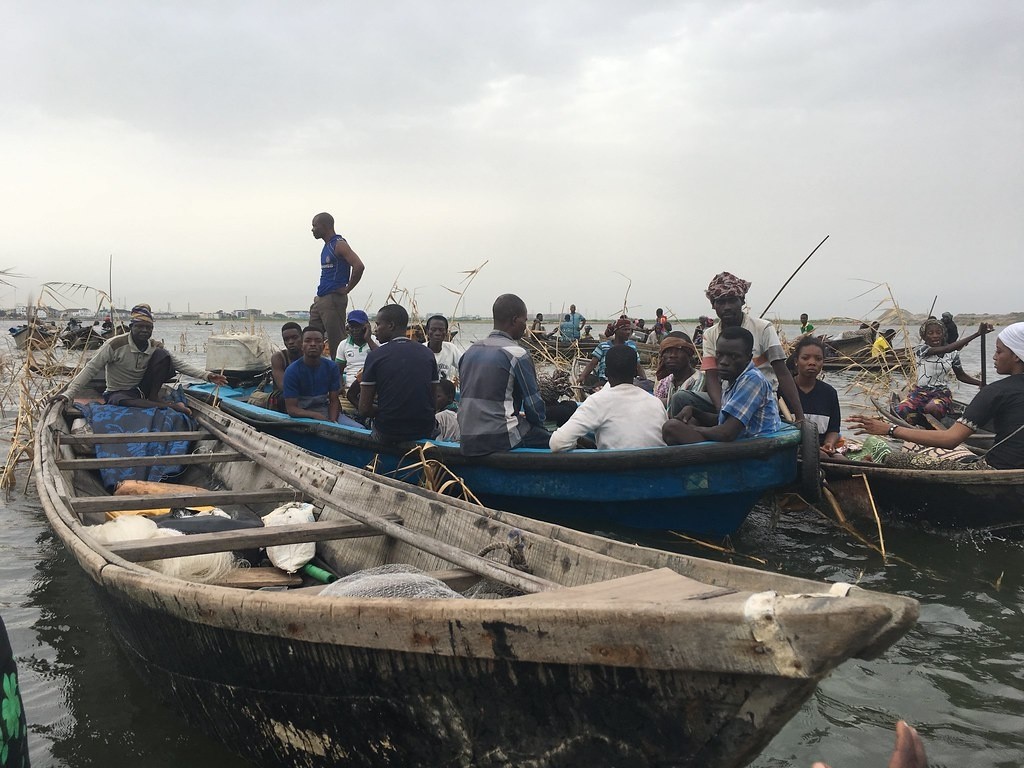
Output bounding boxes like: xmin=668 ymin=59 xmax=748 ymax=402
xmin=205 ymin=335 xmax=265 ymax=371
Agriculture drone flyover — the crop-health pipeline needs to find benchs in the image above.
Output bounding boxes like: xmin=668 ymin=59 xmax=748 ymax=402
xmin=100 ymin=514 xmax=404 ymax=563
xmin=70 ymin=486 xmax=313 ymax=514
xmin=65 ymin=397 xmax=106 ymax=416
xmin=54 ymin=430 xmax=227 ymax=444
xmin=55 ymin=450 xmax=267 ymax=472
xmin=285 ymin=568 xmax=482 ymax=597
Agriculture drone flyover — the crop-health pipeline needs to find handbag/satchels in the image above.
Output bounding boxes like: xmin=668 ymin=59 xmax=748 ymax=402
xmin=248 ymin=376 xmax=282 ymax=413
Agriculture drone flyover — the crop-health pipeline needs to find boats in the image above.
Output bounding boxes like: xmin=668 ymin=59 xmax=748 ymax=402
xmin=195 ymin=323 xmax=213 ymax=325
xmin=101 ymin=323 xmax=130 ymax=339
xmin=33 ymin=376 xmax=923 ymax=768
xmin=796 ymin=432 xmax=1024 ymax=536
xmin=178 ymin=334 xmax=805 ymax=544
xmin=60 ymin=326 xmax=103 ymax=348
xmin=405 ymin=326 xmax=457 ymax=343
xmin=789 ymin=334 xmax=923 ymax=370
xmin=519 ymin=335 xmax=703 ymax=365
xmin=9 ymin=327 xmax=57 ymax=350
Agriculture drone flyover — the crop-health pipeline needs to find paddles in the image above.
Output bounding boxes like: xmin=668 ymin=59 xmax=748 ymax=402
xmin=109 ymin=254 xmax=115 ymax=336
xmin=919 ymin=295 xmax=938 ymax=344
xmin=980 ymin=322 xmax=987 ymax=388
xmin=758 ymin=234 xmax=830 ymax=318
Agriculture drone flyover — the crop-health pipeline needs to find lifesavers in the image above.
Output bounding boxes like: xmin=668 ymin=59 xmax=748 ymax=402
xmin=802 ymin=419 xmax=821 ymax=500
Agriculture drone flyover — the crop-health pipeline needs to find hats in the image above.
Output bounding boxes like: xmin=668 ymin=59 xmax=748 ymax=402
xmin=102 ymin=318 xmax=111 ymax=321
xmin=347 ymin=309 xmax=368 ymax=325
xmin=128 ymin=304 xmax=155 ymax=324
xmin=705 ymin=272 xmax=752 ymax=303
xmin=998 ymin=321 xmax=1024 ymax=364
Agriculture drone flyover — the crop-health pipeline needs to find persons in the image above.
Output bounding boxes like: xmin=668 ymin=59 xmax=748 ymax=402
xmin=800 ymin=313 xmax=814 ymax=334
xmin=549 ymin=318 xmax=669 ymax=453
xmin=929 ymin=312 xmax=959 ymax=344
xmin=271 ymin=293 xmax=552 ymax=455
xmin=657 ymin=272 xmax=848 ymax=462
xmin=619 ymin=308 xmax=713 ymax=348
xmin=844 ymin=321 xmax=1024 ymax=470
xmin=49 ymin=303 xmax=228 ymax=414
xmin=309 ymin=212 xmax=365 ymax=360
xmin=62 ymin=316 xmax=114 ymax=336
xmin=559 ymin=304 xmax=594 ymax=342
xmin=857 ymin=320 xmax=895 ymax=360
xmin=896 ymin=319 xmax=995 ymax=424
xmin=531 ymin=313 xmax=547 ymax=341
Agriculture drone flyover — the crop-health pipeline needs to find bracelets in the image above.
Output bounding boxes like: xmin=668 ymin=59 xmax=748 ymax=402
xmin=888 ymin=425 xmax=898 ymax=439
